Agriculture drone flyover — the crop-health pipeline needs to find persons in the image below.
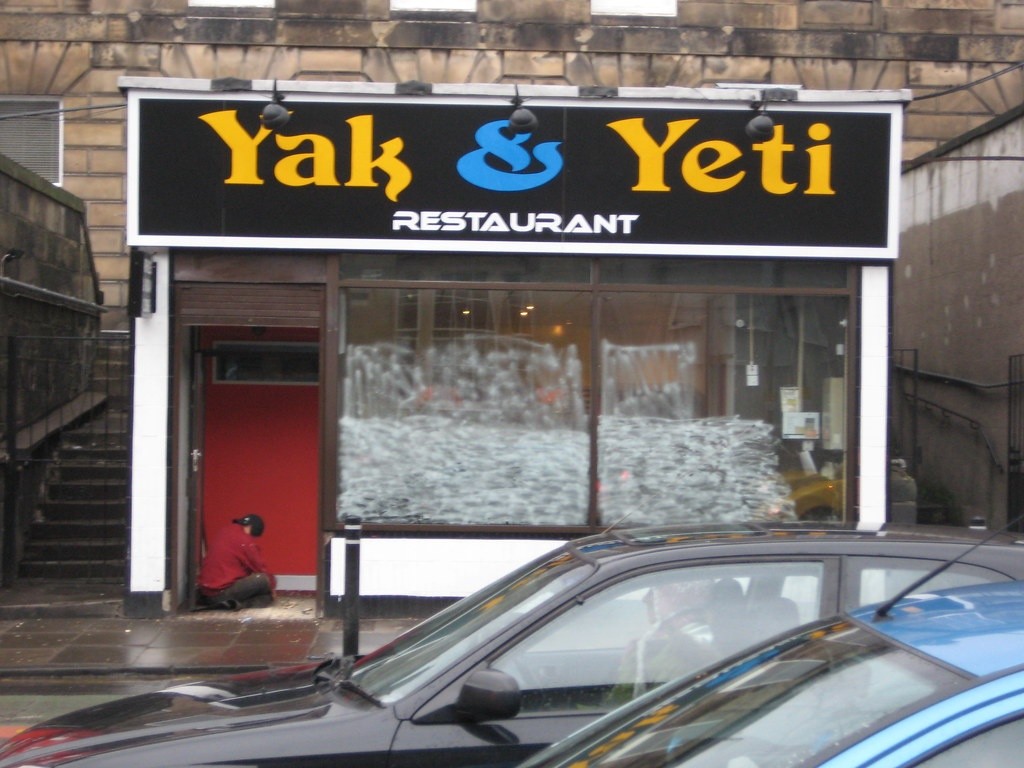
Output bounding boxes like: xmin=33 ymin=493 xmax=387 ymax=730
xmin=196 ymin=514 xmax=278 ymax=611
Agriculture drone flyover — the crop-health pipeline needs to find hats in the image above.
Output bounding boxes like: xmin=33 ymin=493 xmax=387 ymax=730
xmin=233 ymin=514 xmax=264 ymax=531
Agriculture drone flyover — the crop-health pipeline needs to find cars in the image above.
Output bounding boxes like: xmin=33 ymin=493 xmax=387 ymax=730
xmin=1 ymin=523 xmax=1024 ymax=768
xmin=517 ymin=577 xmax=1024 ymax=767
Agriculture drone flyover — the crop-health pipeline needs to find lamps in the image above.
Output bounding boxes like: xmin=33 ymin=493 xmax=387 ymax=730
xmin=261 ymin=78 xmax=289 ymax=130
xmin=509 ymin=84 xmax=538 ymax=133
xmin=745 ymin=90 xmax=775 ymax=142
